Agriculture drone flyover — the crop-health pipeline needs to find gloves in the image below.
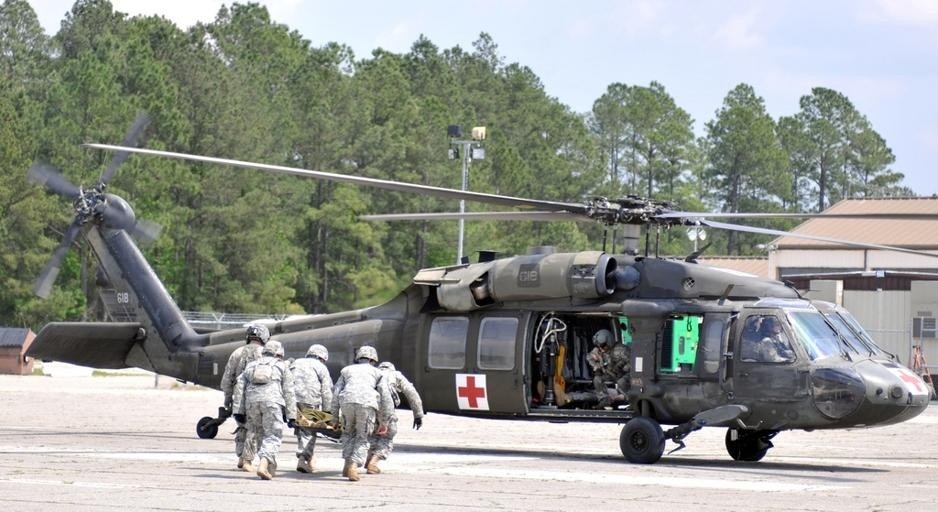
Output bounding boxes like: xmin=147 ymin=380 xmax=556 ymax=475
xmin=412 ymin=418 xmax=423 ymax=431
xmin=286 ymin=418 xmax=296 ymax=429
xmin=234 ymin=415 xmax=246 ymax=424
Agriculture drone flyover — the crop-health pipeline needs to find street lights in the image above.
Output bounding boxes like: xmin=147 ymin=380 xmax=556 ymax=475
xmin=448 ymin=117 xmax=491 ymax=263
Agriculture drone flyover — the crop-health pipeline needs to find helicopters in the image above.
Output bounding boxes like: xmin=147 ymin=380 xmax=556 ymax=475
xmin=11 ymin=101 xmax=938 ymax=475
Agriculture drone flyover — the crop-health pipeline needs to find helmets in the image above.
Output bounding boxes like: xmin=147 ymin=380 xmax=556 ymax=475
xmin=354 ymin=345 xmax=380 ymax=364
xmin=376 ymin=361 xmax=395 ymax=370
xmin=306 ymin=344 xmax=328 ymax=363
xmin=261 ymin=341 xmax=284 ymax=357
xmin=243 ymin=324 xmax=270 ymax=345
xmin=590 ymin=329 xmax=614 ymax=349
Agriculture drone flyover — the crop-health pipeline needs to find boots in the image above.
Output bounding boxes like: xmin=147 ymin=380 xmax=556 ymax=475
xmin=237 ymin=457 xmax=277 ymax=481
xmin=343 ymin=453 xmax=382 ymax=483
xmin=295 ymin=455 xmax=315 ymax=475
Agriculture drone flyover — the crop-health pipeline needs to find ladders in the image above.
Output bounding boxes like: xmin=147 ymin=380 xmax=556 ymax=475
xmin=912 ymin=345 xmax=937 ymax=400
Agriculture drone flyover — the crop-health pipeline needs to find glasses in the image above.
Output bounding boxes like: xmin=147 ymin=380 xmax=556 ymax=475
xmin=596 ymin=342 xmax=606 ymax=349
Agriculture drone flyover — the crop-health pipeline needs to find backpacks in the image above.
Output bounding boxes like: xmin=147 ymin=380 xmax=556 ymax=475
xmin=251 ymin=357 xmax=279 ymax=385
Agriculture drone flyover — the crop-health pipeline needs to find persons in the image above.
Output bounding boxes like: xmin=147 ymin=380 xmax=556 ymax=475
xmin=220 ymin=320 xmax=270 ymax=467
xmin=330 ymin=346 xmax=394 ymax=483
xmin=363 ymin=360 xmax=423 ymax=475
xmin=231 ymin=340 xmax=298 ymax=481
xmin=289 ymin=344 xmax=334 ymax=474
xmin=587 ymin=328 xmax=632 ymax=410
xmin=756 ymin=316 xmax=796 ymax=362
